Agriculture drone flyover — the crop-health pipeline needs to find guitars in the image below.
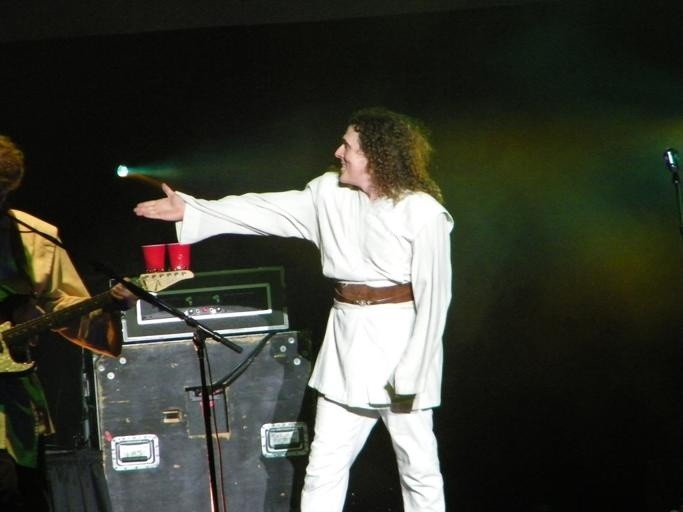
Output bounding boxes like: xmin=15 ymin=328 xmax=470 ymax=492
xmin=1 ymin=266 xmax=194 ymax=373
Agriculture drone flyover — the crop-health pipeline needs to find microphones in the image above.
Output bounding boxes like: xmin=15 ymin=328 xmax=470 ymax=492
xmin=663 ymin=148 xmax=679 ymax=174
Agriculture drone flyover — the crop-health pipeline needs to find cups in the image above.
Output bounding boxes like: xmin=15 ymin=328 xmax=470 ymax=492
xmin=141 ymin=242 xmax=192 ymax=273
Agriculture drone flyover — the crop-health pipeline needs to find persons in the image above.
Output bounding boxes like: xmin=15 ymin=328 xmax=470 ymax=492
xmin=0 ymin=134 xmax=138 ymax=512
xmin=132 ymin=105 xmax=456 ymax=512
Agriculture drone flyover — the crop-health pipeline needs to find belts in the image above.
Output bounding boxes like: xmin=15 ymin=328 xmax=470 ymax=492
xmin=333 ymin=288 xmax=412 ymax=306
